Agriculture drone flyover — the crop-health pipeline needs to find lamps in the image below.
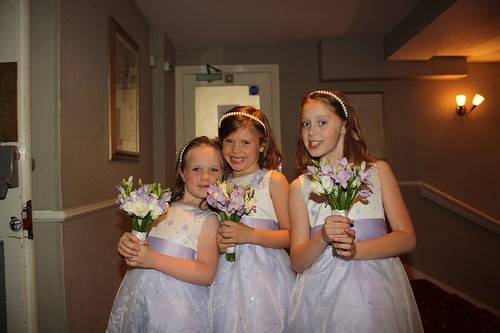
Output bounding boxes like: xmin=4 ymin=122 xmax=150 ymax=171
xmin=456 ymin=94 xmax=485 ymax=115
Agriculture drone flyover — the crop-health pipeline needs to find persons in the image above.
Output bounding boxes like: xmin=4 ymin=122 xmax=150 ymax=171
xmin=205 ymin=105 xmax=291 ymax=333
xmin=289 ymin=88 xmax=425 ymax=333
xmin=106 ymin=135 xmax=230 ymax=333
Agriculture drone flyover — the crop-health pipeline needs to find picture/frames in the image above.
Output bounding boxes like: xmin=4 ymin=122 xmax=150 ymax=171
xmin=107 ymin=15 xmax=142 ymax=161
xmin=342 ymin=91 xmax=387 ymax=160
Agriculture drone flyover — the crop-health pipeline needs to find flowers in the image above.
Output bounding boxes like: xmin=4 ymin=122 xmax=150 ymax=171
xmin=306 ymin=157 xmax=375 ymax=257
xmin=116 ymin=175 xmax=172 ymax=269
xmin=207 ymin=179 xmax=258 ymax=263
